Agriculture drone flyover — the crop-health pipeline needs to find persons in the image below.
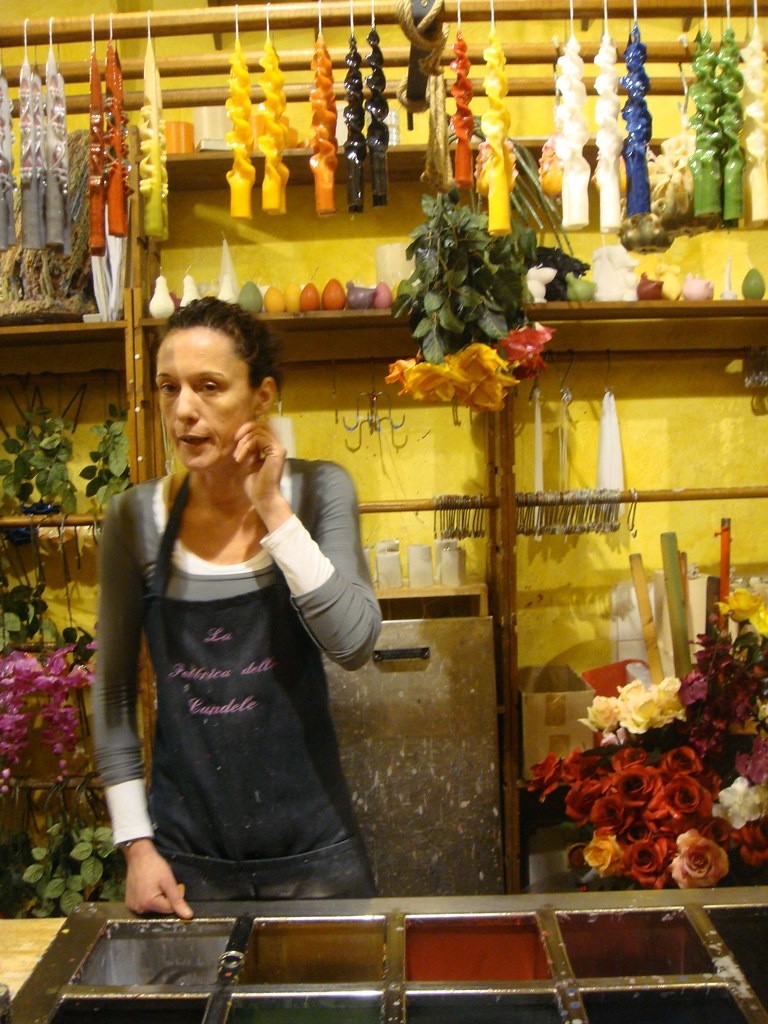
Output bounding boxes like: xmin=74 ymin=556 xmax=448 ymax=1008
xmin=93 ymin=296 xmax=381 ymax=922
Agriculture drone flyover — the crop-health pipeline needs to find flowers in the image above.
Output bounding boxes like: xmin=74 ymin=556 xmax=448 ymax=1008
xmin=0 ymin=378 xmax=132 ymax=761
xmin=384 ymin=114 xmax=589 ymax=413
xmin=513 ymin=587 xmax=767 ymax=891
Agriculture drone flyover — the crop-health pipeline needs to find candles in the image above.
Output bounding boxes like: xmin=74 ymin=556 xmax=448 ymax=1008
xmin=362 ymin=540 xmax=468 ymax=590
xmin=149 ymin=233 xmax=390 ymax=318
xmin=0 ymin=0 xmax=768 ymax=258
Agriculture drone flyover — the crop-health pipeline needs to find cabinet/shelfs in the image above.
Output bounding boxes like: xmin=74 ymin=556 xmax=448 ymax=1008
xmin=0 ymin=140 xmax=767 ymax=899
